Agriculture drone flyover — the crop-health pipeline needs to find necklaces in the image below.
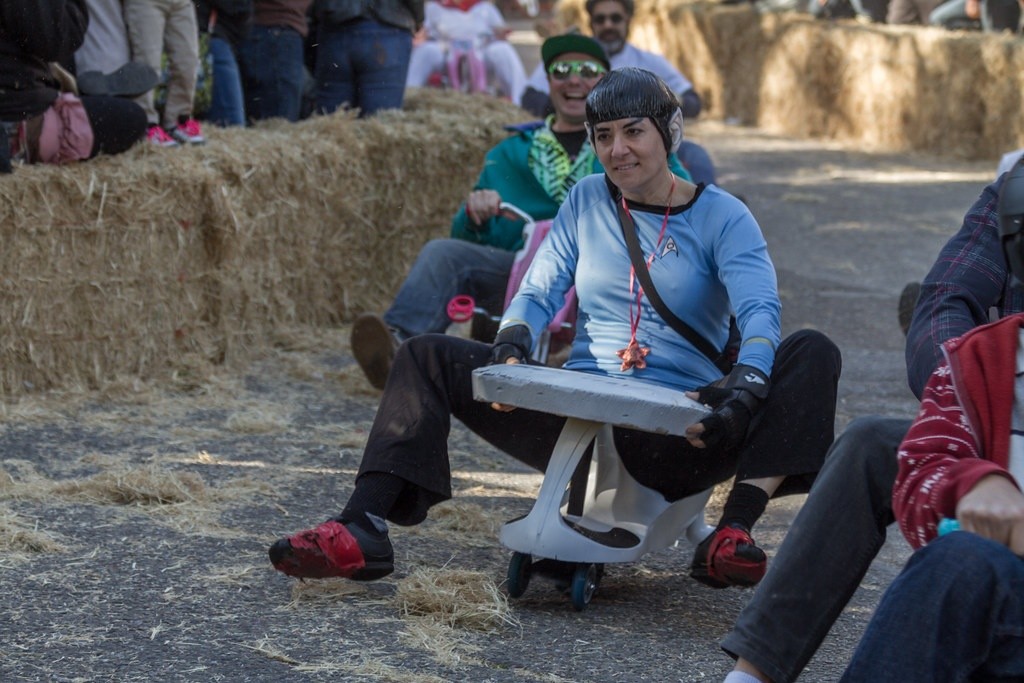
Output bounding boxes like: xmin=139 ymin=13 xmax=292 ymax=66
xmin=617 ymin=169 xmax=676 ymax=371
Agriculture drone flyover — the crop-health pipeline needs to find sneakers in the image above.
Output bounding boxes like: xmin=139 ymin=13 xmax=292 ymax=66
xmin=268 ymin=514 xmax=394 ymax=581
xmin=689 ymin=522 xmax=766 ymax=589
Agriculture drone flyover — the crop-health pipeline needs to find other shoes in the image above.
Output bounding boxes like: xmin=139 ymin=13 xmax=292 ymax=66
xmin=140 ymin=125 xmax=177 ymax=146
xmin=351 ymin=314 xmax=400 ymax=390
xmin=77 ymin=62 xmax=158 ymax=95
xmin=169 ymin=119 xmax=204 ymax=143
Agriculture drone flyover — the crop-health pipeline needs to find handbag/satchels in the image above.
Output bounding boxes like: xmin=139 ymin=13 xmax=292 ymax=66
xmin=155 ymin=31 xmax=213 ymax=115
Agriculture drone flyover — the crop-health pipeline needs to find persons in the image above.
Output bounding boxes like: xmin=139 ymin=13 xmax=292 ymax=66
xmin=725 ymin=0 xmax=1021 ymax=38
xmin=721 ymin=155 xmax=1023 ymax=683
xmin=0 ymin=0 xmax=748 ymax=403
xmin=267 ymin=66 xmax=842 ymax=590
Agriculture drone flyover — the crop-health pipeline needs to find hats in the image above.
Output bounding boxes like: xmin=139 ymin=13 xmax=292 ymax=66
xmin=541 ymin=33 xmax=611 ymax=73
xmin=584 ymin=68 xmax=684 ymax=154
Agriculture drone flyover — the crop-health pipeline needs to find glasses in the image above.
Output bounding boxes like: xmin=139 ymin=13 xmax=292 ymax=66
xmin=592 ymin=13 xmax=631 ymax=24
xmin=547 ymin=58 xmax=607 ymax=79
